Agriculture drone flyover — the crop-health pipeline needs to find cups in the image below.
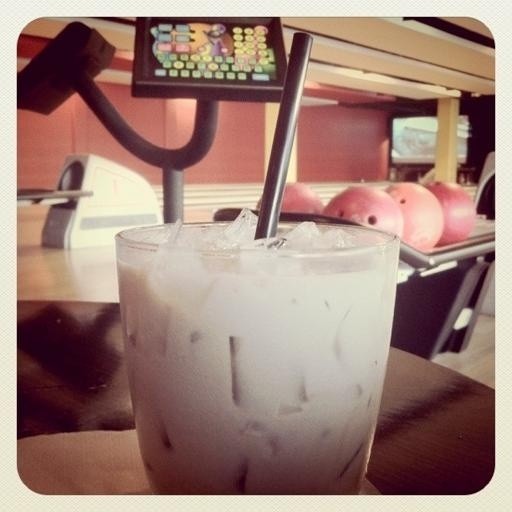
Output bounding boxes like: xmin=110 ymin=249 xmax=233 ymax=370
xmin=116 ymin=219 xmax=402 ymax=495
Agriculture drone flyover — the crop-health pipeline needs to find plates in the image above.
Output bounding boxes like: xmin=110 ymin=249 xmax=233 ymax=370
xmin=17 ymin=429 xmax=379 ymax=494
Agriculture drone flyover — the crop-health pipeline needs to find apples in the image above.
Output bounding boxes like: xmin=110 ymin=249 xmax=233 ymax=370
xmin=323 ymin=185 xmax=405 ymax=239
xmin=381 ymin=181 xmax=445 ymax=252
xmin=256 ymin=181 xmax=325 ymax=217
xmin=423 ymin=178 xmax=477 ymax=248
